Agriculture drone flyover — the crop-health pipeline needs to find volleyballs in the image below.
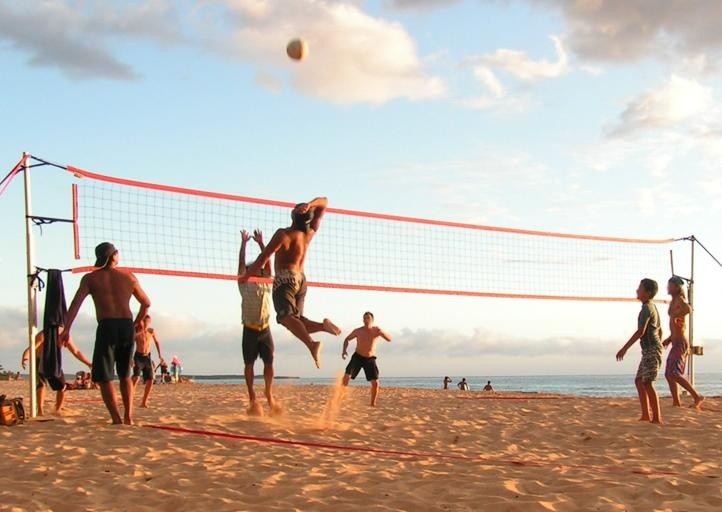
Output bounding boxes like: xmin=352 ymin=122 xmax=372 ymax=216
xmin=287 ymin=40 xmax=307 ymax=60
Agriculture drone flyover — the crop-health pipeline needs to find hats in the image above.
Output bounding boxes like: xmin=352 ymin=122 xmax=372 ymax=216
xmin=94 ymin=242 xmax=115 ymax=269
xmin=295 ymin=203 xmax=314 ymax=232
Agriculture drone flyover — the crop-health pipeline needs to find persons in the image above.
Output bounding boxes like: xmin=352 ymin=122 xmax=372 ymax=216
xmin=614 ymin=278 xmax=665 ymax=425
xmin=154 ymin=359 xmax=168 ymax=383
xmin=341 ymin=311 xmax=392 ymax=407
xmin=132 ymin=315 xmax=167 ymax=408
xmin=483 ymin=381 xmax=494 ymax=392
xmin=15 ymin=372 xmax=21 ymax=380
xmin=22 ymin=326 xmax=91 ymax=417
xmin=58 ymin=241 xmax=151 ymax=425
xmin=443 ymin=375 xmax=452 ymax=389
xmin=239 ymin=227 xmax=279 ymax=416
xmin=662 ymin=276 xmax=703 ymax=409
xmin=457 ymin=378 xmax=468 ymax=390
xmin=65 ymin=373 xmax=99 ymax=390
xmin=7 ymin=371 xmax=13 ymax=381
xmin=240 ymin=195 xmax=342 ymax=370
xmin=171 ymin=356 xmax=182 ymax=383
